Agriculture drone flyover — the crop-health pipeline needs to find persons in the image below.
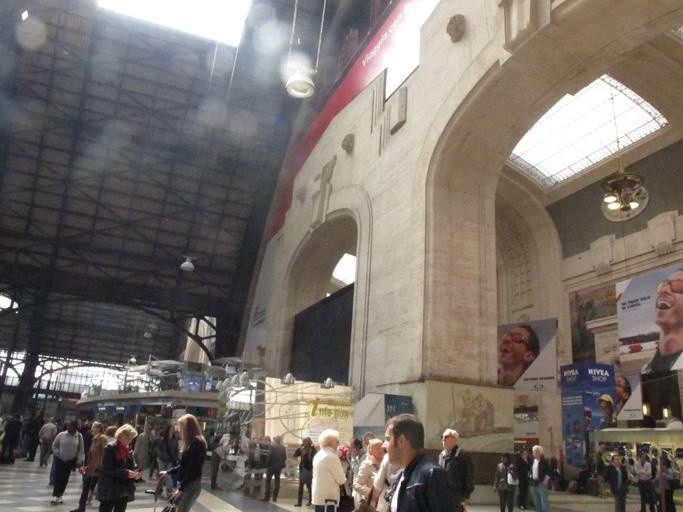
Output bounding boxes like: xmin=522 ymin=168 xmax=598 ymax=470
xmin=492 ymin=445 xmax=683 ymax=511
xmin=615 ymin=376 xmax=633 ymax=414
xmin=597 ymin=393 xmax=615 ymax=428
xmin=666 ymin=398 xmax=683 ymax=429
xmin=498 ymin=324 xmax=539 ymax=387
xmin=640 ymin=267 xmax=682 ymax=375
xmin=2 ymin=412 xmax=474 ymax=512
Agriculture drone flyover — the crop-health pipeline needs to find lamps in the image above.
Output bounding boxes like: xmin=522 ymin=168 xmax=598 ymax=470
xmin=180 ymin=257 xmax=194 ymax=272
xmin=602 ymin=70 xmax=643 ymax=210
xmin=281 ymin=0 xmax=325 ymax=100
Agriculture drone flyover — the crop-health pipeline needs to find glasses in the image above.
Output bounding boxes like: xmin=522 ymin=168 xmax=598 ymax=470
xmin=440 ymin=435 xmax=453 ymax=440
xmin=655 ymin=278 xmax=683 ymax=295
xmin=502 ymin=330 xmax=533 ymax=348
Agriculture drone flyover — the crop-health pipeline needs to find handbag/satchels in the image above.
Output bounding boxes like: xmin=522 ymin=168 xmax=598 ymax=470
xmin=338 ymin=496 xmax=354 ymax=511
xmin=506 ymin=473 xmax=520 ymax=485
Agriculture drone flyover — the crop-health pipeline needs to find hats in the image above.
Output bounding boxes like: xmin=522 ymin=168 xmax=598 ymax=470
xmin=596 ymin=394 xmax=614 ymax=410
